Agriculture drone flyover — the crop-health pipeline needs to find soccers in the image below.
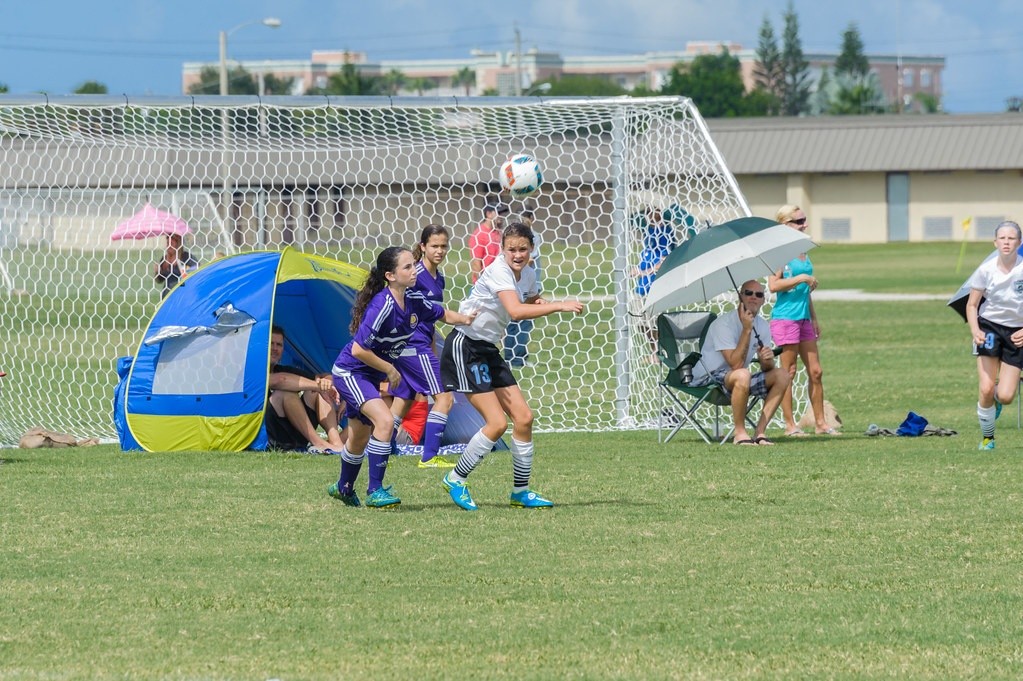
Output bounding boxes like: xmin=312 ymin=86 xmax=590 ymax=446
xmin=499 ymin=154 xmax=544 ymax=198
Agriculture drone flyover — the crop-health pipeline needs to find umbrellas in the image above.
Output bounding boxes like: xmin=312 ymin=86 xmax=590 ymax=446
xmin=111 ymin=195 xmax=192 ymax=264
xmin=642 ymin=217 xmax=822 ymax=350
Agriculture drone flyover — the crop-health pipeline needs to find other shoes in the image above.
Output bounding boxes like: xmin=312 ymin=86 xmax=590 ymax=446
xmin=994 ymin=401 xmax=1003 ymax=419
xmin=979 ymin=437 xmax=995 ymax=451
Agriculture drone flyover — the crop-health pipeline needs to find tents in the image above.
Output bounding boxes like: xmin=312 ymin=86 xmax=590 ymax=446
xmin=113 ymin=245 xmax=510 ymax=452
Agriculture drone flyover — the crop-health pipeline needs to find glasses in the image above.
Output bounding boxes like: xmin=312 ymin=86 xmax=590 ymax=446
xmin=741 ymin=290 xmax=765 ymax=298
xmin=166 ymin=234 xmax=179 ymax=241
xmin=788 ymin=216 xmax=807 ymax=225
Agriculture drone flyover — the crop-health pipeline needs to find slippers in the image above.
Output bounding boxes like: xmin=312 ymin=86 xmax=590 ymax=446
xmin=732 ymin=437 xmax=759 ymax=448
xmin=755 ymin=437 xmax=772 ymax=445
xmin=817 ymin=427 xmax=842 ymax=437
xmin=785 ymin=430 xmax=809 ymax=438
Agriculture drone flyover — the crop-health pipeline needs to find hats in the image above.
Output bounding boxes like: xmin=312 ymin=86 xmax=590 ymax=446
xmin=483 ymin=203 xmax=508 ymax=214
xmin=516 ymin=204 xmax=534 ymax=219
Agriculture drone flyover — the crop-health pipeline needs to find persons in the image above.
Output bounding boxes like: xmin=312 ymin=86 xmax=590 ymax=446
xmin=468 ymin=202 xmax=509 ymax=284
xmin=234 ymin=324 xmax=345 ymax=453
xmin=327 ymin=246 xmax=481 ymax=504
xmin=689 ymin=279 xmax=790 ymax=445
xmin=155 ymin=233 xmax=201 ymax=302
xmin=966 ymin=221 xmax=1023 ymax=450
xmin=326 ymin=377 xmax=428 ymax=446
xmin=633 ymin=206 xmax=679 ymax=423
xmin=387 ymin=224 xmax=458 ymax=468
xmin=440 ymin=223 xmax=584 ymax=511
xmin=946 ymin=240 xmax=1023 ymax=429
xmin=769 ymin=207 xmax=841 ymax=437
xmin=504 ymin=204 xmax=540 ymax=367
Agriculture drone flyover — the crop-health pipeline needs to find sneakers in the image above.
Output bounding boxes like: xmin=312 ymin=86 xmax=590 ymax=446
xmin=418 ymin=457 xmax=456 ymax=469
xmin=509 ymin=489 xmax=553 ymax=510
xmin=328 ymin=481 xmax=362 ymax=508
xmin=441 ymin=473 xmax=477 ymax=510
xmin=366 ymin=484 xmax=401 ymax=510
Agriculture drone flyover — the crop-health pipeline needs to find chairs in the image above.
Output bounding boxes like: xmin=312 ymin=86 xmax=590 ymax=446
xmin=658 ymin=311 xmax=763 ymax=446
